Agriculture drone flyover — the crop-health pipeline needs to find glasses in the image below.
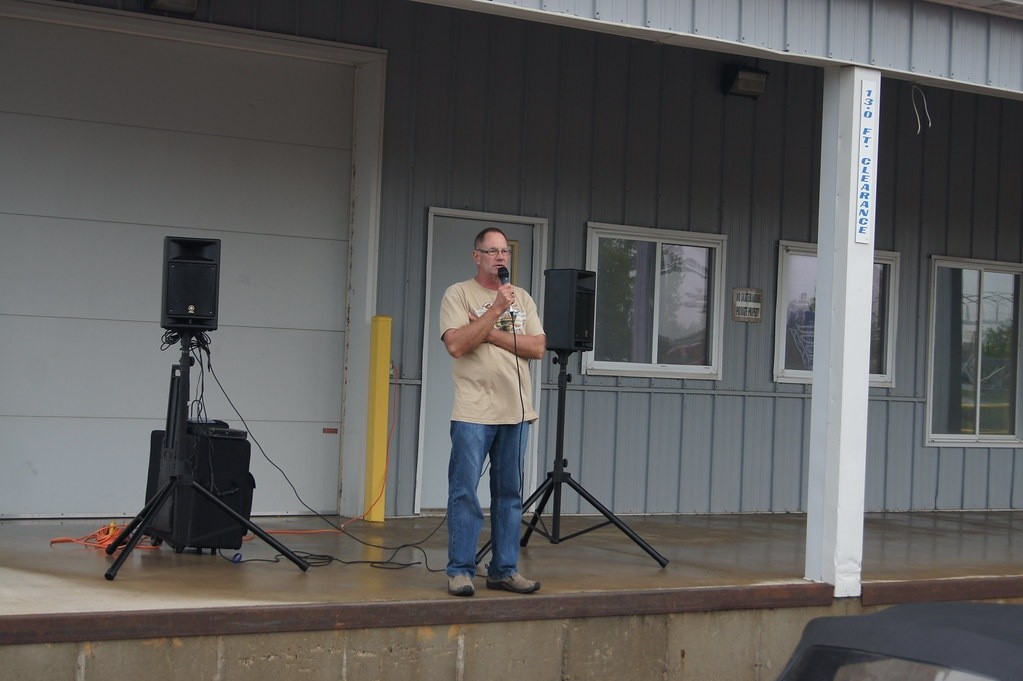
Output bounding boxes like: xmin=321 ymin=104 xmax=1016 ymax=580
xmin=476 ymin=247 xmax=513 ymax=256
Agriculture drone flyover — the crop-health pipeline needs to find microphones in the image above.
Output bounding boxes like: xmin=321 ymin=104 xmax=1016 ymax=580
xmin=498 ymin=266 xmax=513 ymax=315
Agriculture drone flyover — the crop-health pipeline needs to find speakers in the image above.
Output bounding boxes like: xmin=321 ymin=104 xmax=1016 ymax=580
xmin=144 ymin=430 xmax=252 ymax=550
xmin=544 ymin=268 xmax=597 ymax=351
xmin=161 ymin=236 xmax=221 ymax=332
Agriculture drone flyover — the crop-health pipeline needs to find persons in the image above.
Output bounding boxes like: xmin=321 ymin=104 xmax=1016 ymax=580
xmin=439 ymin=227 xmax=547 ymax=595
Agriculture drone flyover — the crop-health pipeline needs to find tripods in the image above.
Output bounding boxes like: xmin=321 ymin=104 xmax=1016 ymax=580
xmin=475 ymin=350 xmax=670 ymax=569
xmin=105 ymin=329 xmax=309 ymax=581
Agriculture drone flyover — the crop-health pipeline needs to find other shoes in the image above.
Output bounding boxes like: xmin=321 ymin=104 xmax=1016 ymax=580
xmin=448 ymin=575 xmax=475 ymax=596
xmin=484 ymin=564 xmax=541 ymax=593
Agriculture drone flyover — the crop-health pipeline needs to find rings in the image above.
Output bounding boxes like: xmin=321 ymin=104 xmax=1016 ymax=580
xmin=511 ymin=293 xmax=514 ymax=298
